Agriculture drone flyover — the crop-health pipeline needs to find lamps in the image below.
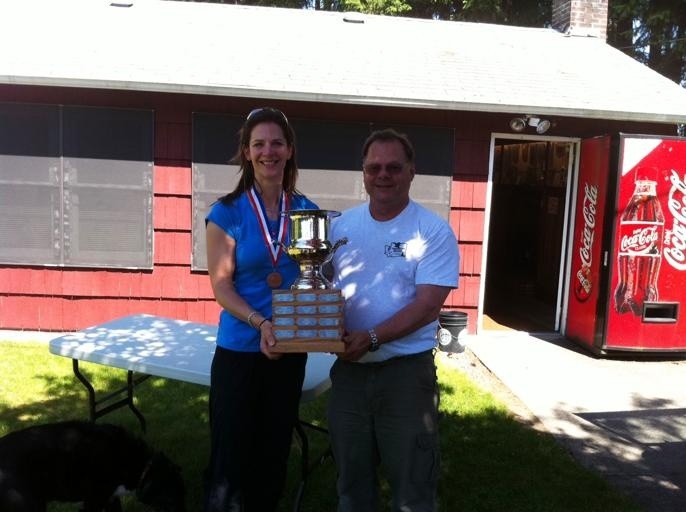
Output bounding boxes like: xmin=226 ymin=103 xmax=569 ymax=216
xmin=509 ymin=114 xmax=551 ymax=134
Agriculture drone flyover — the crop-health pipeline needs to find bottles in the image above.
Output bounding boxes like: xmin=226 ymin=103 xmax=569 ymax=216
xmin=613 ymin=168 xmax=664 ymax=317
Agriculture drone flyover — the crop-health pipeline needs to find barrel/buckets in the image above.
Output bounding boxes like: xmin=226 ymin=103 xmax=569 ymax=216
xmin=437 ymin=310 xmax=468 ymax=354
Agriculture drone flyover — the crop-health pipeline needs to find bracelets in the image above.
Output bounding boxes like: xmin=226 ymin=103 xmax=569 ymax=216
xmin=257 ymin=318 xmax=271 ymax=331
xmin=368 ymin=327 xmax=380 ymax=352
xmin=248 ymin=312 xmax=261 ymax=328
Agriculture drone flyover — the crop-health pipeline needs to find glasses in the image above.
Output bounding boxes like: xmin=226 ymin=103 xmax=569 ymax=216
xmin=245 ymin=105 xmax=289 ymax=128
xmin=364 ymin=161 xmax=403 ymax=176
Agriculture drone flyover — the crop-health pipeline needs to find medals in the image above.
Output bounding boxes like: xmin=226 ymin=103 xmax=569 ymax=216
xmin=266 ymin=270 xmax=284 ymax=289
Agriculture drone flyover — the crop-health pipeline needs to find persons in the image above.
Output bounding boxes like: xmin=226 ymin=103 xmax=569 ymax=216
xmin=326 ymin=127 xmax=460 ymax=511
xmin=202 ymin=105 xmax=323 ymax=512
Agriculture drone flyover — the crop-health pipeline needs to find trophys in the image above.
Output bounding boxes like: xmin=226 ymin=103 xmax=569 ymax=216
xmin=270 ymin=208 xmax=348 ymax=289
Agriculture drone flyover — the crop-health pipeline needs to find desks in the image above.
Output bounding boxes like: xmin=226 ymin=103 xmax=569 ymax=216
xmin=49 ymin=314 xmax=339 ymax=468
